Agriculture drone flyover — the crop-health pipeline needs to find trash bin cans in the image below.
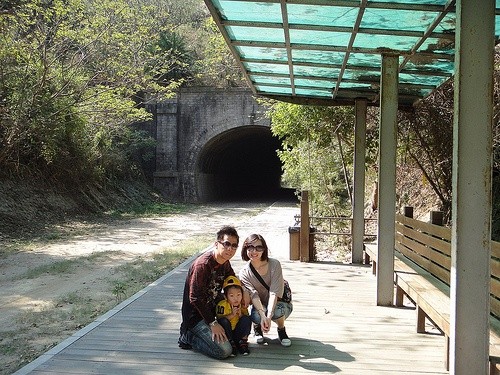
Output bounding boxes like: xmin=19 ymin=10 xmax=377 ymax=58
xmin=289 ymin=227 xmax=316 ymax=260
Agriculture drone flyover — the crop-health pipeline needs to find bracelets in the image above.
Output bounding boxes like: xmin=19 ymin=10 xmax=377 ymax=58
xmin=209 ymin=319 xmax=218 ymax=326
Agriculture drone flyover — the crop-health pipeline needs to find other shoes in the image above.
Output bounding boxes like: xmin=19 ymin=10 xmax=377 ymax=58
xmin=238 ymin=339 xmax=249 ymax=357
xmin=230 ymin=339 xmax=238 ymax=356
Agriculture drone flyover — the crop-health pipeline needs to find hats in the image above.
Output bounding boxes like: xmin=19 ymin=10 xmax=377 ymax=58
xmin=223 ymin=276 xmax=241 ymax=290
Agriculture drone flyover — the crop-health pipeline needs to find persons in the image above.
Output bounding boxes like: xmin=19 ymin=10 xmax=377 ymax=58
xmin=177 ymin=226 xmax=252 ymax=360
xmin=238 ymin=234 xmax=293 ymax=346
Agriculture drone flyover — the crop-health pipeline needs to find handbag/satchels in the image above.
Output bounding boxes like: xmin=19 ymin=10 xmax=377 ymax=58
xmin=278 ymin=280 xmax=291 ymax=304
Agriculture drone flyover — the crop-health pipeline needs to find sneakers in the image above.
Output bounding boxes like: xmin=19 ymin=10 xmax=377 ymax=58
xmin=178 ymin=322 xmax=192 ymax=349
xmin=277 ymin=326 xmax=292 ymax=346
xmin=253 ymin=323 xmax=264 ymax=343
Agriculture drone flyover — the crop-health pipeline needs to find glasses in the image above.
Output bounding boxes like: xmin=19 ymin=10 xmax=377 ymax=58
xmin=246 ymin=245 xmax=266 ymax=252
xmin=218 ymin=241 xmax=239 ymax=250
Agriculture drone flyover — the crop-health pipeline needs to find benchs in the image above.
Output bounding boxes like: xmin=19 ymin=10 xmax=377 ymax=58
xmin=365 ymin=212 xmax=499 ymax=375
